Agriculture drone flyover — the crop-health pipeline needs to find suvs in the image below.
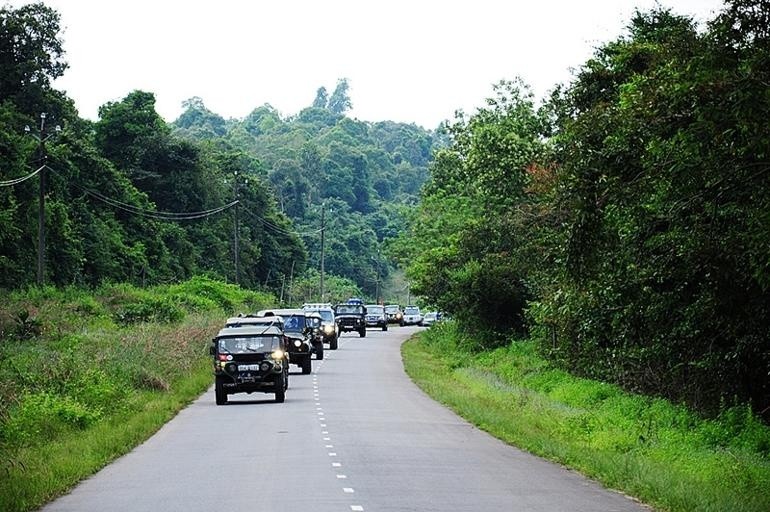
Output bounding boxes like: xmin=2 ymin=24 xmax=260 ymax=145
xmin=209 ymin=298 xmax=448 ymax=406
